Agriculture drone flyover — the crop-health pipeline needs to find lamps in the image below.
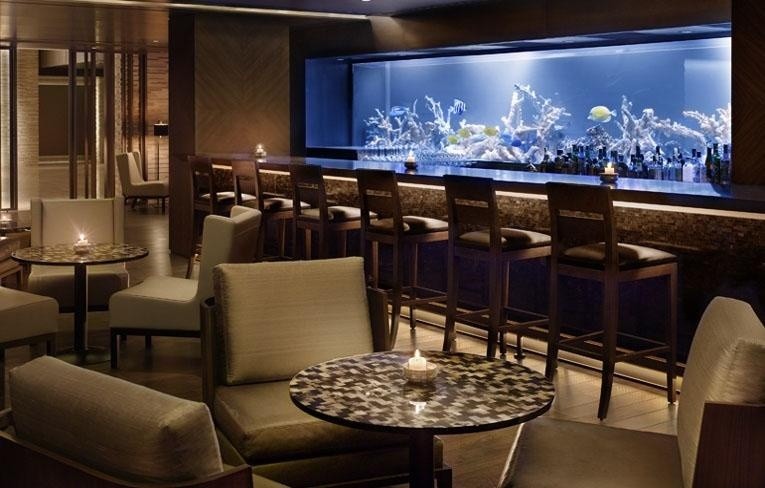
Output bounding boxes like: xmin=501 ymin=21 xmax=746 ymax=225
xmin=152 ymin=120 xmax=169 ymax=208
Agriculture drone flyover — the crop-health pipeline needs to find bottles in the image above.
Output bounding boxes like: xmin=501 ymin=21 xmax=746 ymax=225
xmin=538 ymin=142 xmax=730 ymax=183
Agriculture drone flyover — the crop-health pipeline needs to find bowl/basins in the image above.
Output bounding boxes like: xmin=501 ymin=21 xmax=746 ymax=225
xmin=252 ymin=150 xmax=269 ymax=159
xmin=403 ymin=161 xmax=420 ymax=171
xmin=599 ymin=173 xmax=620 ymax=184
xmin=401 ymin=360 xmax=439 ymax=384
xmin=71 ymin=243 xmax=96 ymax=254
